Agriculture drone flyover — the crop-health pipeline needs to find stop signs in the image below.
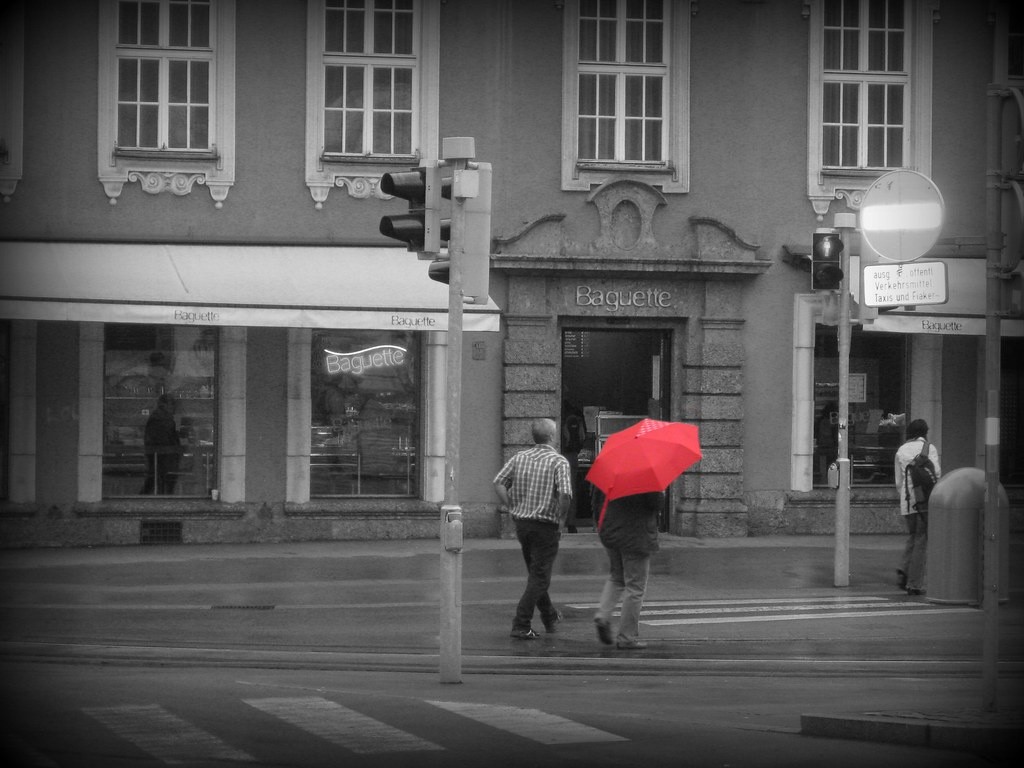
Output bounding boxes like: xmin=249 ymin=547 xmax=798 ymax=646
xmin=860 ymin=170 xmax=945 ymax=266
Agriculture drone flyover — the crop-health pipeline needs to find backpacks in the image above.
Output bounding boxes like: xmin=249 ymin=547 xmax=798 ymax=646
xmin=905 ymin=443 xmax=937 ymax=524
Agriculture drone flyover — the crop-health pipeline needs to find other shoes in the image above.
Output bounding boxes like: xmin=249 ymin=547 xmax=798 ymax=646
xmin=594 ymin=615 xmax=613 ymax=644
xmin=896 ymin=569 xmax=907 ymax=590
xmin=545 ymin=609 xmax=563 ymax=633
xmin=617 ymin=639 xmax=648 ymax=650
xmin=510 ymin=627 xmax=540 ymax=639
xmin=908 ymin=589 xmax=926 ymax=595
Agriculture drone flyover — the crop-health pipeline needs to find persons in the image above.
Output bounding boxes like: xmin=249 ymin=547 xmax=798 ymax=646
xmin=140 ymin=394 xmax=182 ymax=497
xmin=561 ymin=415 xmax=586 ymax=533
xmin=592 ymin=485 xmax=665 ymax=650
xmin=895 ymin=419 xmax=942 ymax=595
xmin=493 ymin=417 xmax=573 ymax=639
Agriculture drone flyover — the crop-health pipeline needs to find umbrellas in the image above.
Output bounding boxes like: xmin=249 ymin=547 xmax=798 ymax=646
xmin=585 ymin=418 xmax=703 ymax=501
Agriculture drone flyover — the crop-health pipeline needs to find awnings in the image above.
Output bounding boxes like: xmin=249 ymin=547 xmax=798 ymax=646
xmin=806 ymin=256 xmax=1024 ymax=336
xmin=0 ymin=241 xmax=501 ymax=332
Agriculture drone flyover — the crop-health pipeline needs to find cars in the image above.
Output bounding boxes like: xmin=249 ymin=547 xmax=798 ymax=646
xmin=817 ymin=400 xmax=902 ymax=482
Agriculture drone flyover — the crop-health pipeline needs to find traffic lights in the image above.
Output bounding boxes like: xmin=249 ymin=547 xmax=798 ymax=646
xmin=811 ymin=232 xmax=845 ymax=289
xmin=428 ymin=176 xmax=452 ymax=285
xmin=380 ymin=165 xmax=429 ymax=257
